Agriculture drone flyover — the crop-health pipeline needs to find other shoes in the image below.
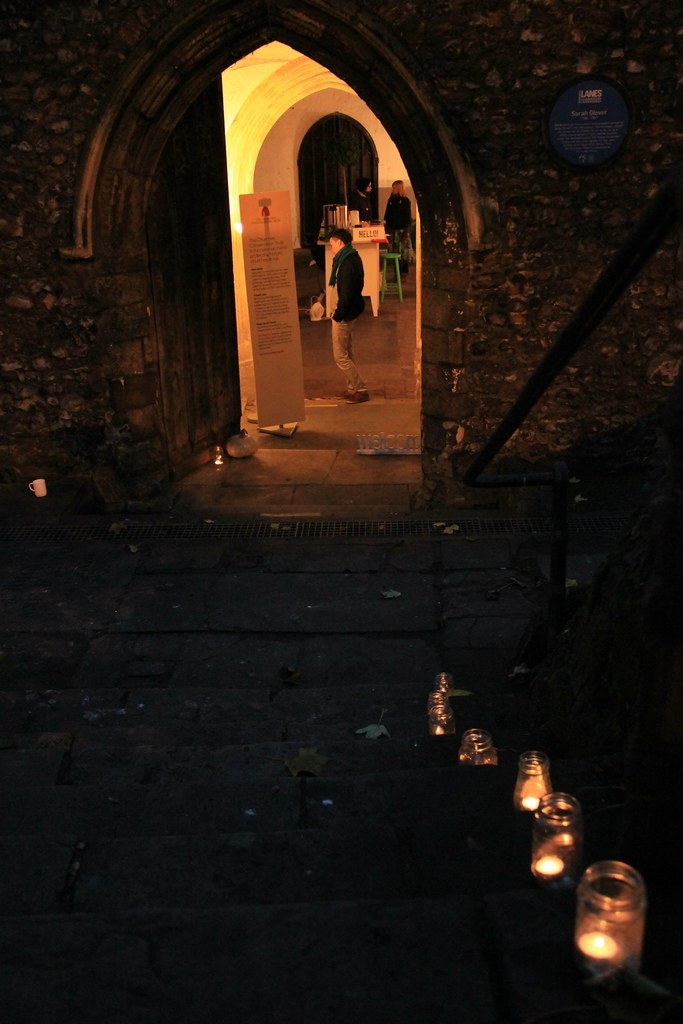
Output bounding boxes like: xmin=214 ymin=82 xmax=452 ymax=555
xmin=392 ymin=273 xmax=408 ymax=282
xmin=337 ymin=389 xmax=369 ymax=404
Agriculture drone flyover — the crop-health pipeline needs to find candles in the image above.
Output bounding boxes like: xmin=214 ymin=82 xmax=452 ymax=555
xmin=427 ymin=673 xmax=616 ymax=963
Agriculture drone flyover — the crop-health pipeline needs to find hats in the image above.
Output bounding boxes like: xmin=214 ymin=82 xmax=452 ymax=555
xmin=355 ymin=178 xmax=370 ymax=191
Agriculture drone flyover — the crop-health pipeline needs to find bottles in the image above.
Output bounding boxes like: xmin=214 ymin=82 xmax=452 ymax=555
xmin=458 ymin=729 xmax=497 ymax=786
xmin=426 ymin=672 xmax=456 ymax=735
xmin=512 ymin=751 xmax=554 ymax=814
xmin=573 ymin=860 xmax=646 ymax=975
xmin=530 ymin=792 xmax=582 ymax=881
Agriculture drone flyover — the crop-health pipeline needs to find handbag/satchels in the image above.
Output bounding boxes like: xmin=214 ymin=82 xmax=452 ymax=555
xmin=401 ymin=238 xmax=416 ymax=263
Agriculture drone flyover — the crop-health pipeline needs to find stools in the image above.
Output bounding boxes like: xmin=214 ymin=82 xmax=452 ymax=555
xmin=382 ymin=253 xmax=404 ymax=302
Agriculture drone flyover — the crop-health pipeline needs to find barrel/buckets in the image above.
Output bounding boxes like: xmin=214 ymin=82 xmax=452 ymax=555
xmin=322 ymin=204 xmax=347 ymax=236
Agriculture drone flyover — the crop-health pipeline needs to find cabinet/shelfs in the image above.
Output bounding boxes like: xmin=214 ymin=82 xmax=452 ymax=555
xmin=317 ymin=223 xmax=386 ymax=317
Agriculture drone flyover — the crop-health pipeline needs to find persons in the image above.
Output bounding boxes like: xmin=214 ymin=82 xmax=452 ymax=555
xmin=348 ymin=178 xmax=373 ymax=221
xmin=384 ymin=180 xmax=411 ymax=283
xmin=329 ymin=229 xmax=370 ymax=403
